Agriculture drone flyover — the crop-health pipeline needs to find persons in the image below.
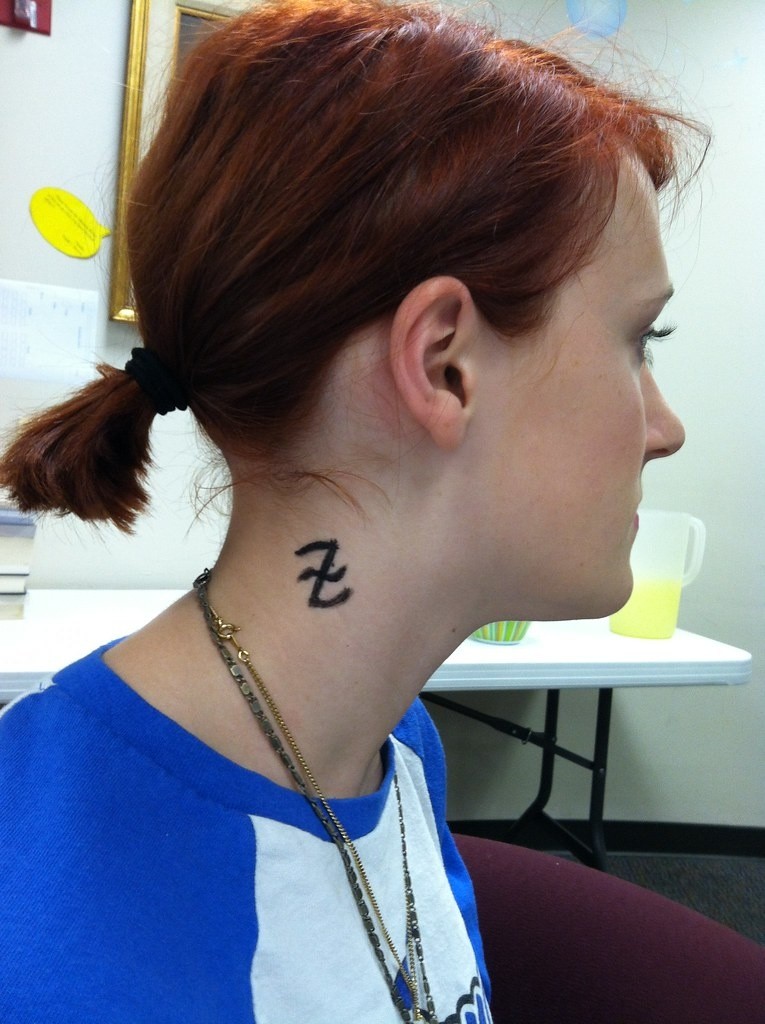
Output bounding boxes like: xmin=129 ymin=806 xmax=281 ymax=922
xmin=0 ymin=1 xmax=708 ymax=1024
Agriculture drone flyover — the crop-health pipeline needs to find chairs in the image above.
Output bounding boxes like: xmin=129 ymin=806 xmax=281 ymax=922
xmin=447 ymin=831 xmax=765 ymax=1024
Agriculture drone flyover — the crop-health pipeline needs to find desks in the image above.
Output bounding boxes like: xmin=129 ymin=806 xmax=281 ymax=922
xmin=0 ymin=588 xmax=753 ymax=880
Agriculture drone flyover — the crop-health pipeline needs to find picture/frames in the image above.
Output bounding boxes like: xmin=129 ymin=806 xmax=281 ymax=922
xmin=109 ymin=0 xmax=276 ymax=324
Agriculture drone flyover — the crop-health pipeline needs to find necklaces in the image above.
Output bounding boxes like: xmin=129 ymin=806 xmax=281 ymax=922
xmin=193 ymin=567 xmax=439 ymax=1024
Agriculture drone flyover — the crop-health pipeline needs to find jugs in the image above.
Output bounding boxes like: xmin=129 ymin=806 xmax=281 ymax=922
xmin=610 ymin=508 xmax=706 ymax=640
